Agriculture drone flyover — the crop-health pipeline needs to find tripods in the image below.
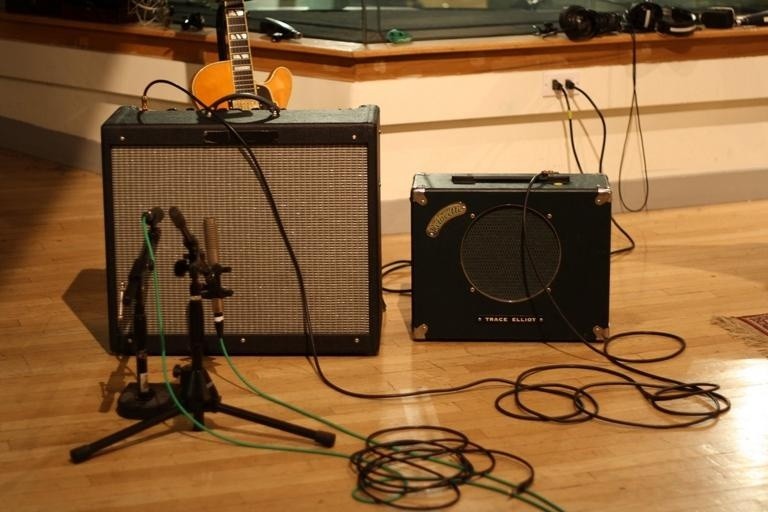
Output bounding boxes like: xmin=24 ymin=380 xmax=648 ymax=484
xmin=70 ymin=285 xmax=336 ymax=464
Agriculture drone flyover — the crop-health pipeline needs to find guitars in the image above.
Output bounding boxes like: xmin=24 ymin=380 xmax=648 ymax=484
xmin=191 ymin=0 xmax=293 ymax=111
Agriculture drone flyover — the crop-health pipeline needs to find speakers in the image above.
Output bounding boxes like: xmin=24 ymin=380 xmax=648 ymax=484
xmin=100 ymin=106 xmax=380 ymax=356
xmin=409 ymin=172 xmax=612 ymax=342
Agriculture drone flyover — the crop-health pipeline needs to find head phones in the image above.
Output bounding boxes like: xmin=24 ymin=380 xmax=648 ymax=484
xmin=559 ymin=5 xmax=619 ymax=43
xmin=622 ymin=1 xmax=697 ymax=34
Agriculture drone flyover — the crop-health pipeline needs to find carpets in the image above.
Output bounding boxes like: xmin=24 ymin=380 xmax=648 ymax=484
xmin=711 ymin=312 xmax=767 ymax=355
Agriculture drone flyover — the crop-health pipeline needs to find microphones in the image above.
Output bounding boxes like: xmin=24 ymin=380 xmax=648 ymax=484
xmin=203 ymin=216 xmax=226 ymax=337
xmin=140 ymin=206 xmax=165 ymax=228
xmin=167 ymin=207 xmax=205 ymax=263
